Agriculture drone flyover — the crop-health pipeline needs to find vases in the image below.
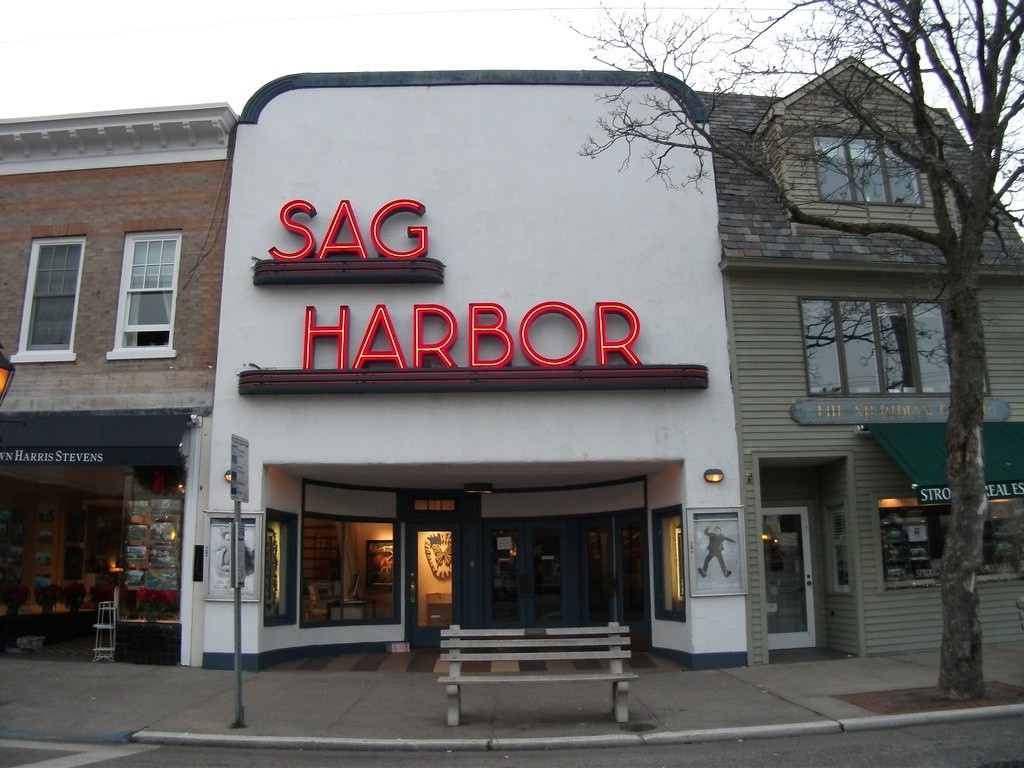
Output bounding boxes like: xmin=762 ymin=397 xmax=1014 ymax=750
xmin=43 ymin=603 xmax=53 ymax=612
xmin=9 ymin=606 xmax=20 ymax=615
xmin=71 ymin=606 xmax=80 ymax=612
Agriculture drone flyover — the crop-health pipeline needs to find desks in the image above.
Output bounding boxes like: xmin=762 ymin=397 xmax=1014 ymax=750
xmin=343 ymin=600 xmax=368 ymax=620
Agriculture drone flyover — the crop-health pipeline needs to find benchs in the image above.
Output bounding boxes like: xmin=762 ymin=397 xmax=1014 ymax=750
xmin=437 ymin=623 xmax=640 ymax=727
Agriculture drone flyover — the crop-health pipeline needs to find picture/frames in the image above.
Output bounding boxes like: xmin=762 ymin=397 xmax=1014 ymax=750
xmin=203 ymin=510 xmax=264 ymax=602
xmin=686 ymin=505 xmax=748 ymax=597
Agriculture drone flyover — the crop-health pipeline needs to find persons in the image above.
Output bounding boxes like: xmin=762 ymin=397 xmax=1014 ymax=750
xmin=697 ymin=524 xmax=736 ymax=579
xmin=217 ymin=529 xmax=231 ymax=578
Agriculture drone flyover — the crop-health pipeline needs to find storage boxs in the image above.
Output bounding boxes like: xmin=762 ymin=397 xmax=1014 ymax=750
xmin=385 ymin=642 xmax=411 ymax=653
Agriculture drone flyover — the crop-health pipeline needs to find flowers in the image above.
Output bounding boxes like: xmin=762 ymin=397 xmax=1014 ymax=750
xmin=37 ymin=583 xmax=86 ymax=610
xmin=0 ymin=581 xmax=29 ymax=607
xmin=138 ymin=587 xmax=179 ymax=622
xmin=88 ymin=584 xmax=119 ymax=609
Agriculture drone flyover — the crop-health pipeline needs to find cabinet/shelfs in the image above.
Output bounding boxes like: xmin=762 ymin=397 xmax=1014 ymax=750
xmin=989 ymin=499 xmax=1024 ymax=573
xmin=880 ymin=506 xmax=932 ymax=581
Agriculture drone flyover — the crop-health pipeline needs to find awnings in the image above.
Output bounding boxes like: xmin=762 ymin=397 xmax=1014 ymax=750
xmin=855 ymin=422 xmax=1024 ymax=506
xmin=0 ymin=411 xmax=195 ymax=466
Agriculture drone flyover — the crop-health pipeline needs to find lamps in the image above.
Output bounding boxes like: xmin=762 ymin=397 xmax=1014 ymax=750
xmin=704 ymin=469 xmax=724 ymax=482
xmin=463 ymin=483 xmax=493 ymax=494
xmin=0 ymin=343 xmax=26 ymax=426
